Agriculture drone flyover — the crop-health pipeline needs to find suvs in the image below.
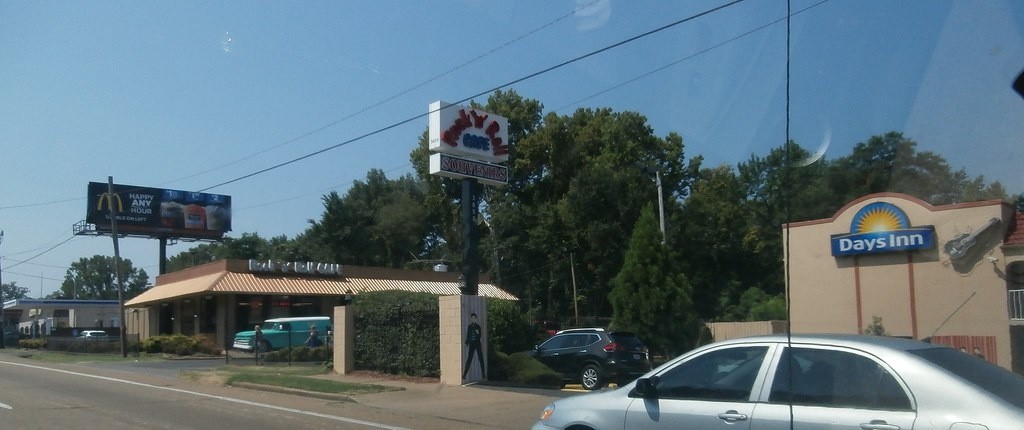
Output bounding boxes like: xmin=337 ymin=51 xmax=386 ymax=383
xmin=522 ymin=327 xmax=654 ymax=391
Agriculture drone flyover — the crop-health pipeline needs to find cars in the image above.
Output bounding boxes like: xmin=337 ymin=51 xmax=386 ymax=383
xmin=233 ymin=316 xmax=334 ymax=355
xmin=529 ymin=332 xmax=1024 ymax=430
xmin=78 ymin=330 xmax=109 ymax=340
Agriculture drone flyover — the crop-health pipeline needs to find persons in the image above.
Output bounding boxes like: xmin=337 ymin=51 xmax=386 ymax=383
xmin=305 ymin=325 xmax=318 ymax=348
xmin=463 ymin=313 xmax=486 ymax=379
xmin=253 ymin=325 xmax=264 ymax=352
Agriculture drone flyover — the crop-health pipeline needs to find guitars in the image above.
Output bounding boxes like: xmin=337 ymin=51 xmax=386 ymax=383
xmin=942 ymin=217 xmax=1001 ymax=260
